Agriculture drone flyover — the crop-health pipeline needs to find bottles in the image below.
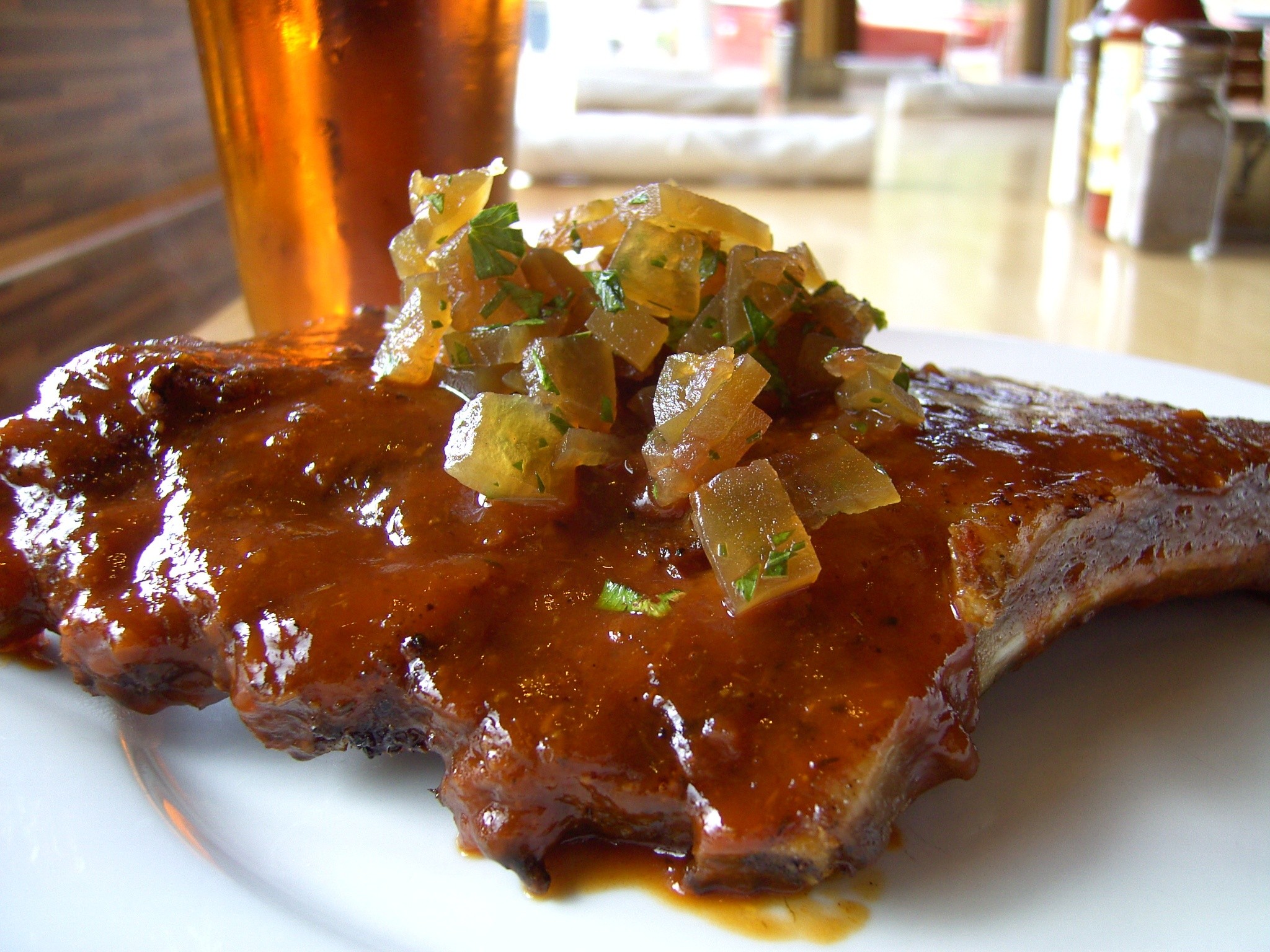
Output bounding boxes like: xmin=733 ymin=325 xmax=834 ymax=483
xmin=1046 ymin=24 xmax=1100 ymax=205
xmin=1101 ymin=22 xmax=1229 ymax=255
xmin=1085 ymin=0 xmax=1210 ymax=231
xmin=1217 ymin=19 xmax=1270 ymax=253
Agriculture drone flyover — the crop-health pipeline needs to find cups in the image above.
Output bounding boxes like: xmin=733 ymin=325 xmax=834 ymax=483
xmin=187 ymin=0 xmax=529 ymax=368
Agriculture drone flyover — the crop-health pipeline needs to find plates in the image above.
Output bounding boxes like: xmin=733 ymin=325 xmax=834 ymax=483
xmin=0 ymin=323 xmax=1270 ymax=948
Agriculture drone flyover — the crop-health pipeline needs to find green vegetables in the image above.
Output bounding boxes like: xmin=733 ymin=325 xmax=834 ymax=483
xmin=421 ymin=177 xmax=911 ymax=615
